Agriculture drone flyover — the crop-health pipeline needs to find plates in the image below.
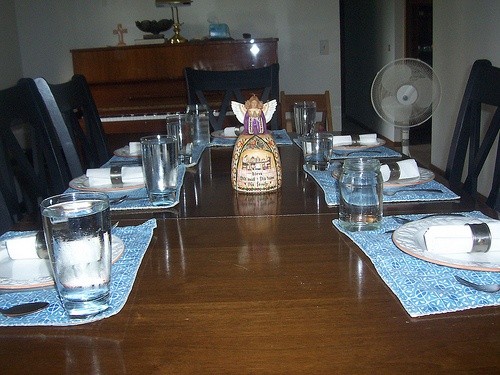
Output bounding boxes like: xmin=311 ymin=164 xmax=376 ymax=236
xmin=113 ymin=147 xmax=143 ymax=158
xmin=331 ymin=166 xmax=436 ymax=187
xmin=210 ymin=127 xmax=240 ymax=139
xmin=332 ymin=137 xmax=387 ymax=150
xmin=391 ymin=217 xmax=500 ymax=272
xmin=0 ymin=234 xmax=125 ymax=290
xmin=69 ymin=174 xmax=145 ymax=192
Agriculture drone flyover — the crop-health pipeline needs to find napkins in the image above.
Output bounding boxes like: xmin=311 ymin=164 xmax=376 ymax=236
xmin=86 ymin=167 xmax=144 ymax=186
xmin=381 ymin=159 xmax=419 ymax=182
xmin=333 ymin=134 xmax=378 ymax=147
xmin=423 ymin=220 xmax=500 ymax=255
xmin=5 ymin=234 xmax=122 ymax=260
xmin=129 ymin=142 xmax=142 ymax=156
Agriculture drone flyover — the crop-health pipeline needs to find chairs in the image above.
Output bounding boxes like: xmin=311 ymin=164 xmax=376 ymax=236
xmin=279 ymin=90 xmax=333 ymax=132
xmin=0 ymin=77 xmax=73 ymax=236
xmin=445 ymin=59 xmax=500 ymax=211
xmin=33 ymin=73 xmax=112 ymax=179
xmin=184 ymin=62 xmax=279 ymax=131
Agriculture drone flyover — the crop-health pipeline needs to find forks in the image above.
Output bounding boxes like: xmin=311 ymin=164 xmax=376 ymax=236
xmin=454 ymin=274 xmax=500 ymax=293
xmin=333 ymin=150 xmax=381 ymax=157
xmin=275 ymin=132 xmax=283 ymax=140
xmin=383 ymin=188 xmax=443 ymax=197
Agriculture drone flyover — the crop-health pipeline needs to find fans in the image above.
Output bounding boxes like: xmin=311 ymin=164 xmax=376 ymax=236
xmin=370 ymin=58 xmax=443 ymax=158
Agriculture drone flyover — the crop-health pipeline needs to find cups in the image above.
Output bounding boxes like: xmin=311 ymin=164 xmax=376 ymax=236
xmin=301 ymin=132 xmax=333 ymax=171
xmin=166 ymin=114 xmax=194 ymax=164
xmin=39 ymin=191 xmax=112 ymax=319
xmin=293 ymin=101 xmax=316 ymax=138
xmin=186 ymin=105 xmax=210 ymax=145
xmin=140 ymin=135 xmax=178 ymax=206
xmin=338 ymin=159 xmax=384 ymax=231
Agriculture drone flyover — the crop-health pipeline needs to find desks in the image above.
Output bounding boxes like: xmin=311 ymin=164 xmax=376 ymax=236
xmin=0 ymin=132 xmax=500 ymax=375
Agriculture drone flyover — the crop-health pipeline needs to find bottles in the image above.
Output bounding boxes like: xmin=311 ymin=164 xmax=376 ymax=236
xmin=231 ymin=189 xmax=282 ymax=266
xmin=230 ymin=95 xmax=283 ymax=193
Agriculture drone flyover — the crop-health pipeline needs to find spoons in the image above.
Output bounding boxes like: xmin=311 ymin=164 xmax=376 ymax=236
xmin=0 ymin=302 xmax=49 ymax=317
xmin=109 ymin=194 xmax=129 ymax=207
xmin=392 ymin=214 xmax=465 ymax=225
